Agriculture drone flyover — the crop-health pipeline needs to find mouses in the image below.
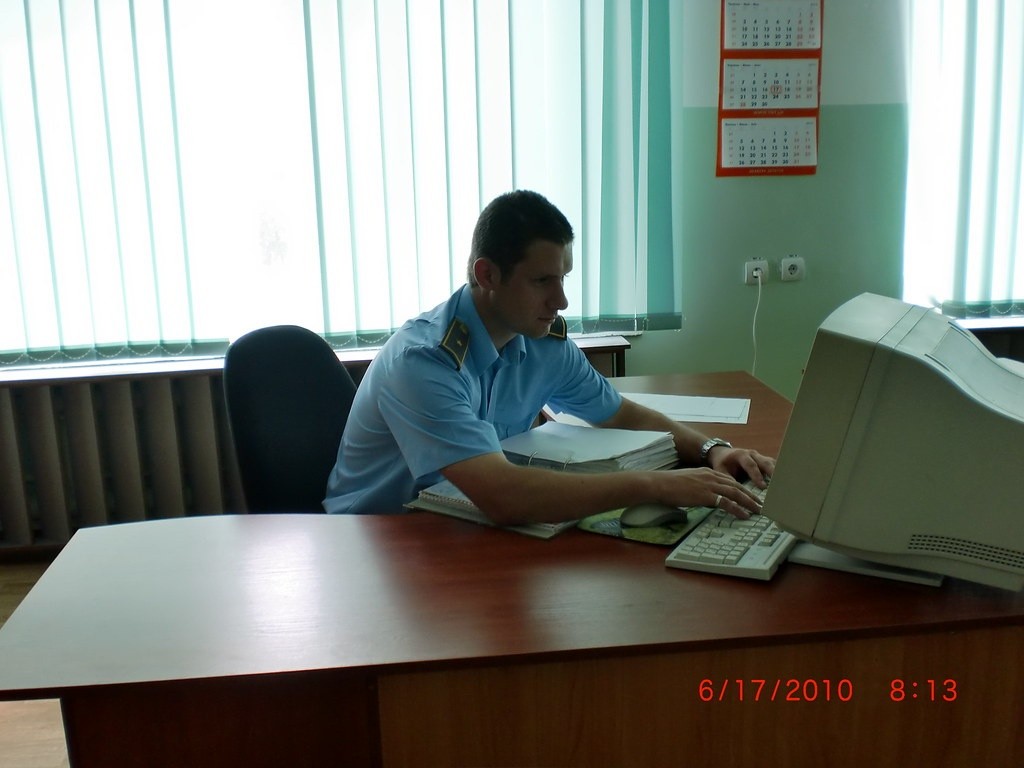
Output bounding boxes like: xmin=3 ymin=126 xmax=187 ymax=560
xmin=620 ymin=505 xmax=687 ymax=528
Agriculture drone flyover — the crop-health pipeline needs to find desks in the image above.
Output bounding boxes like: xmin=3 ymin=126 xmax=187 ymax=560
xmin=0 ymin=370 xmax=1024 ymax=768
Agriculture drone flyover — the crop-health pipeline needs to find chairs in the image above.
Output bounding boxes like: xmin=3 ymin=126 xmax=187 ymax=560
xmin=222 ymin=325 xmax=369 ymax=512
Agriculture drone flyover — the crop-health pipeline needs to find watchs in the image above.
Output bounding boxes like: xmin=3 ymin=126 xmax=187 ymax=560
xmin=701 ymin=438 xmax=733 ymax=459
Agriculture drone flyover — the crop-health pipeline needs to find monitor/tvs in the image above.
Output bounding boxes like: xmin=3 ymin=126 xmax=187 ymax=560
xmin=760 ymin=291 xmax=1024 ymax=592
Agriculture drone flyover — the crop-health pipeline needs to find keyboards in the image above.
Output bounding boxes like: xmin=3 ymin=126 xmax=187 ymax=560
xmin=665 ymin=470 xmax=797 ymax=579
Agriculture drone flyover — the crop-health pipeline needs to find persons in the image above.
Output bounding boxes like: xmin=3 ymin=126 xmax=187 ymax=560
xmin=323 ymin=189 xmax=778 ymax=529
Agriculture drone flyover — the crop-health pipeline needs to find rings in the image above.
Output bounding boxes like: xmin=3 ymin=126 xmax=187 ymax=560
xmin=715 ymin=495 xmax=722 ymax=506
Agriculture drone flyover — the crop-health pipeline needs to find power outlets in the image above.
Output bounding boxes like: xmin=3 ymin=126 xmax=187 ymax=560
xmin=745 ymin=261 xmax=769 ymax=285
xmin=782 ymin=258 xmax=805 ymax=281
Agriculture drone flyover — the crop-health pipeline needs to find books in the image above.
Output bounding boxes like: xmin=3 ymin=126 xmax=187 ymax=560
xmin=417 ymin=478 xmax=568 ymax=532
